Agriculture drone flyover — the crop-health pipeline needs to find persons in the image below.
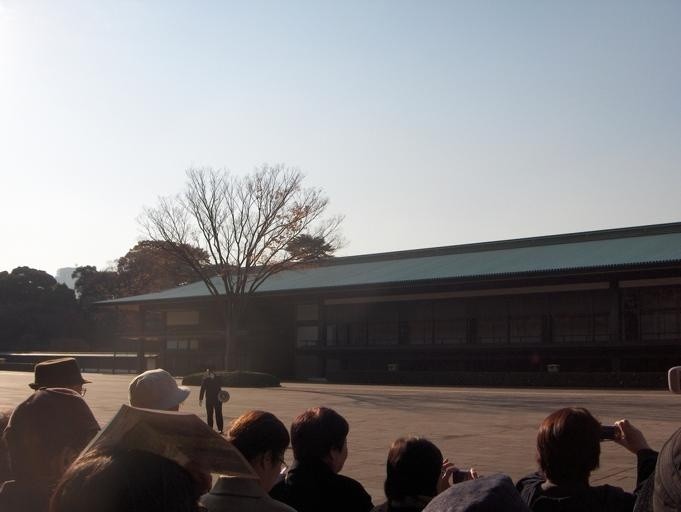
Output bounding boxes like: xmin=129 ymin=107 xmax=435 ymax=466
xmin=1 ymin=357 xmax=374 ymax=511
xmin=373 ymin=357 xmax=681 ymax=511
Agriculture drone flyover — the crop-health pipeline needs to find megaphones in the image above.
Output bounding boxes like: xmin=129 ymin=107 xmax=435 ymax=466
xmin=217 ymin=391 xmax=230 ymax=403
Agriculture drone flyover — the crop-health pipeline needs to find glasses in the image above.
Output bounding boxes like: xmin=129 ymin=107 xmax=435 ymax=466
xmin=276 ymin=456 xmax=288 ymax=473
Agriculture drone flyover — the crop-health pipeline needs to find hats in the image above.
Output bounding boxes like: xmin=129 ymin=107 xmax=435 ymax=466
xmin=30 ymin=359 xmax=93 ymax=388
xmin=422 ymin=474 xmax=529 ymax=511
xmin=4 ymin=386 xmax=101 ymax=437
xmin=130 ymin=369 xmax=190 ymax=409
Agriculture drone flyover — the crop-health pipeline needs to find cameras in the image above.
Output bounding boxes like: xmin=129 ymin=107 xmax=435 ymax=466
xmin=600 ymin=425 xmax=621 ymax=440
xmin=451 ymin=469 xmax=470 ymax=484
